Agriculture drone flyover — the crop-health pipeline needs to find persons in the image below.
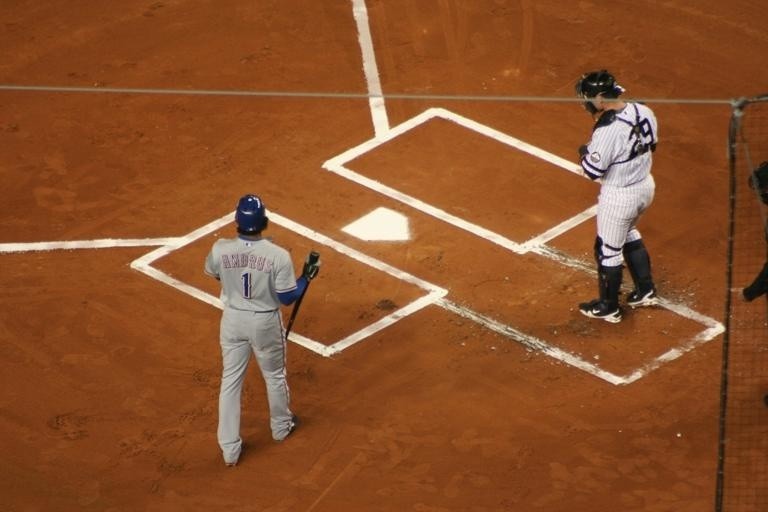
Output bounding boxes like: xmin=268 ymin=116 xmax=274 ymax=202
xmin=574 ymin=67 xmax=662 ymax=327
xmin=736 ymin=155 xmax=768 ymax=307
xmin=201 ymin=192 xmax=321 ymax=468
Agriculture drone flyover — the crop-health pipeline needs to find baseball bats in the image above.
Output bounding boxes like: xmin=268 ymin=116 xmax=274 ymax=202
xmin=286 ymin=252 xmax=319 ymax=339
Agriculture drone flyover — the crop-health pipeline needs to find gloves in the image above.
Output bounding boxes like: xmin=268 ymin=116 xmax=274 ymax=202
xmin=303 ymin=256 xmax=320 ymax=280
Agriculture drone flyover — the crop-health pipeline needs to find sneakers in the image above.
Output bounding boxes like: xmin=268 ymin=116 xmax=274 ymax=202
xmin=272 ymin=415 xmax=298 ymax=442
xmin=626 ymin=286 xmax=657 ymax=306
xmin=579 ymin=299 xmax=623 ymax=324
xmin=224 ymin=460 xmax=237 ymax=467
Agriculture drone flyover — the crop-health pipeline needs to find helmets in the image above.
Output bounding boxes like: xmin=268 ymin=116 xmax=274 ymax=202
xmin=581 ymin=69 xmax=626 ymax=98
xmin=236 ymin=194 xmax=266 ymax=232
